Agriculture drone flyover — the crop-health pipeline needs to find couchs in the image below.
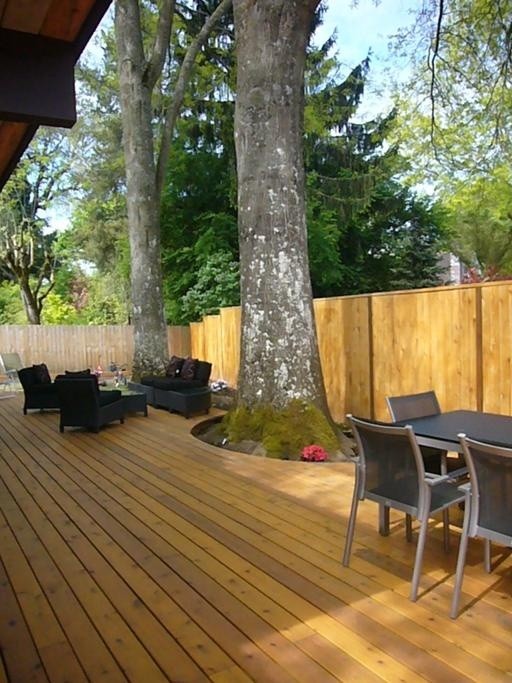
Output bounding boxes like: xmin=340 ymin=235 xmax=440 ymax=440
xmin=155 ymin=360 xmax=213 ymax=419
xmin=33 ymin=364 xmax=54 ymax=392
xmin=59 ymin=378 xmax=124 ymax=433
xmin=16 ymin=367 xmax=63 ymax=414
xmin=128 ymin=356 xmax=185 ymax=407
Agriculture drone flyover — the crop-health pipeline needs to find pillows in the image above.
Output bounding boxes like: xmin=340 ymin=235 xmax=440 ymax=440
xmin=180 ymin=356 xmax=199 ymax=380
xmin=167 ymin=354 xmax=184 ymax=377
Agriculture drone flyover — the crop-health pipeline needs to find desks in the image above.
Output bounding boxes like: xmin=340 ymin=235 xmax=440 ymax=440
xmin=377 ymin=409 xmax=512 ymax=537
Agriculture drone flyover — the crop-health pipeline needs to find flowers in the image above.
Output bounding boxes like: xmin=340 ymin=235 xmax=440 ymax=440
xmin=301 ymin=444 xmax=329 ymax=461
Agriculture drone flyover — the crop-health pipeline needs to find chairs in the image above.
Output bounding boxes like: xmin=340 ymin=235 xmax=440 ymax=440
xmin=449 ymin=432 xmax=512 ymax=619
xmin=384 ymin=390 xmax=469 ymax=554
xmin=65 ymin=368 xmax=90 ymax=375
xmin=0 ymin=352 xmax=26 ymax=393
xmin=54 ymin=374 xmax=121 ymax=406
xmin=341 ymin=413 xmax=492 ymax=601
